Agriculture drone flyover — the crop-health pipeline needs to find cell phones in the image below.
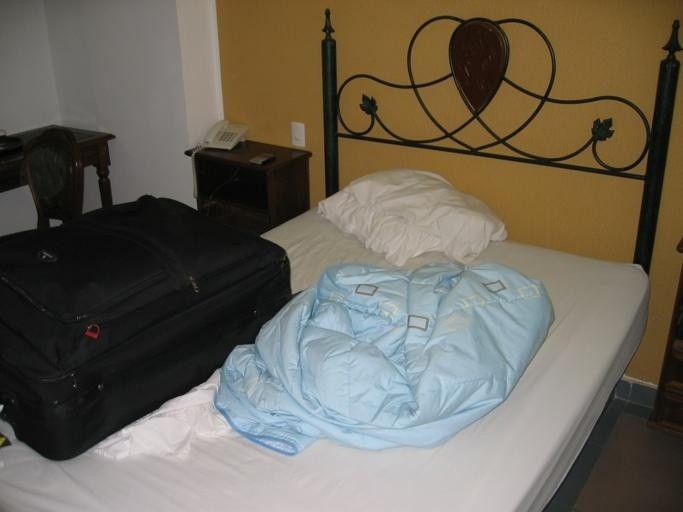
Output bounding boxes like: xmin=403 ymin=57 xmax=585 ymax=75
xmin=249 ymin=153 xmax=274 ymax=166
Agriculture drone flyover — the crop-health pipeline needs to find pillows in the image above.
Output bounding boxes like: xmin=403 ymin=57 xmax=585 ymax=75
xmin=317 ymin=169 xmax=504 ymax=263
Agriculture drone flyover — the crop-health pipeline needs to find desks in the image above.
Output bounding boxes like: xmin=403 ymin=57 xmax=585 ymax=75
xmin=0 ymin=126 xmax=119 ymax=209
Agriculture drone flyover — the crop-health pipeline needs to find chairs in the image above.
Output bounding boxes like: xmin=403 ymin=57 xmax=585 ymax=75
xmin=23 ymin=125 xmax=84 ymax=229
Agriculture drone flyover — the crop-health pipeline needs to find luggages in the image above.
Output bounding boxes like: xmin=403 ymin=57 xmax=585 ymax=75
xmin=0 ymin=193 xmax=293 ymax=460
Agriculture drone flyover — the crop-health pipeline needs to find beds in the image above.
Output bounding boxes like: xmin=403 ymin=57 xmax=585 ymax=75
xmin=0 ymin=7 xmax=678 ymax=511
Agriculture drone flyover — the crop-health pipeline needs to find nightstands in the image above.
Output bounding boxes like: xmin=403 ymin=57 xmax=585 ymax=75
xmin=184 ymin=140 xmax=312 ymax=236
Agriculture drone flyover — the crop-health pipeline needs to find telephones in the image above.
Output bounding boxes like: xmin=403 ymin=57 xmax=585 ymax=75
xmin=200 ymin=119 xmax=249 ymax=152
